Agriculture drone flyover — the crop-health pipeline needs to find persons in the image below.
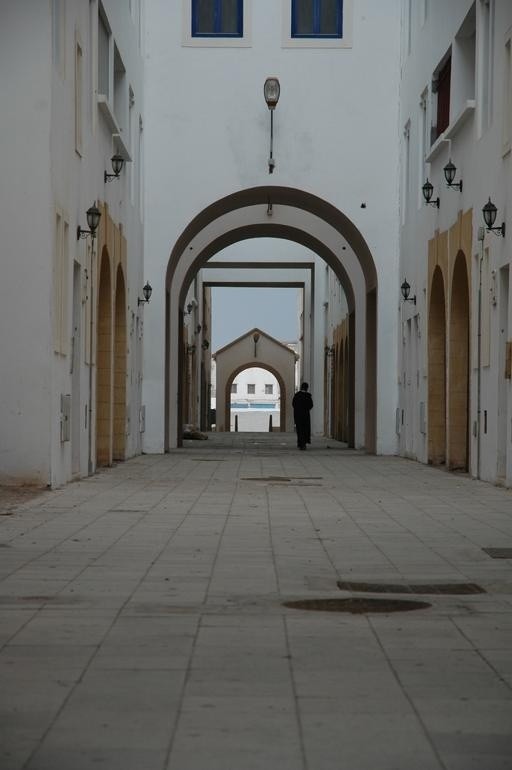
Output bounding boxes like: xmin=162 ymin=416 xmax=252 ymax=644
xmin=292 ymin=381 xmax=314 ymax=451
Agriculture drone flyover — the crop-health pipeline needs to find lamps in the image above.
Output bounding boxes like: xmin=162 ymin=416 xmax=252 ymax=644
xmin=77 ymin=200 xmax=102 ymax=240
xmin=401 ymin=277 xmax=416 ymax=305
xmin=137 ymin=280 xmax=153 ymax=305
xmin=481 ymin=197 xmax=504 ymax=237
xmin=421 ymin=161 xmax=463 ymax=207
xmin=264 ymin=76 xmax=280 ymax=175
xmin=104 ymin=145 xmax=125 ymax=183
xmin=183 ymin=302 xmax=193 ymax=316
